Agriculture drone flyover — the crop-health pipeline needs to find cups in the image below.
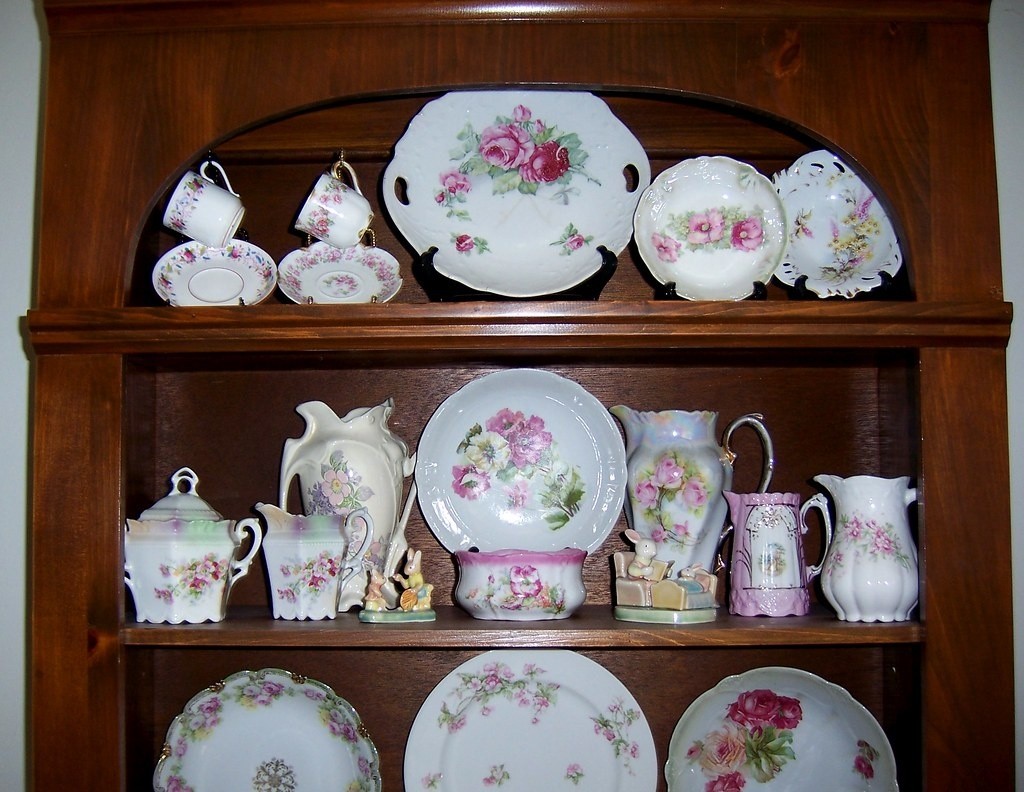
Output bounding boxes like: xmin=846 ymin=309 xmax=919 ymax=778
xmin=163 ymin=160 xmax=246 ymax=249
xmin=295 ymin=161 xmax=373 ymax=249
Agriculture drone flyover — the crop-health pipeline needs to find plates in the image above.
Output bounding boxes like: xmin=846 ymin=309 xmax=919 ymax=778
xmin=665 ymin=666 xmax=900 ymax=792
xmin=405 ymin=650 xmax=657 ymax=792
xmin=774 ymin=150 xmax=902 ymax=298
xmin=384 ymin=91 xmax=651 ymax=298
xmin=152 ymin=238 xmax=277 ymax=306
xmin=633 ymin=155 xmax=789 ymax=301
xmin=415 ymin=368 xmax=629 ymax=556
xmin=153 ymin=669 xmax=381 ymax=792
xmin=277 ymin=241 xmax=403 ymax=305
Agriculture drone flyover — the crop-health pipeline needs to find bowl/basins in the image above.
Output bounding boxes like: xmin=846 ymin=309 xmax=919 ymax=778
xmin=454 ymin=546 xmax=588 ymax=621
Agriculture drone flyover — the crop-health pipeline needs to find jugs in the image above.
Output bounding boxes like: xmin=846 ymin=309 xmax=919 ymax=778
xmin=609 ymin=405 xmax=774 ymax=583
xmin=256 ymin=502 xmax=375 ymax=621
xmin=722 ymin=490 xmax=832 ymax=617
xmin=814 ymin=474 xmax=918 ymax=622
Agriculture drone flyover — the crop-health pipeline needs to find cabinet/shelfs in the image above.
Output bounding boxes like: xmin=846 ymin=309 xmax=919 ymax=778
xmin=15 ymin=0 xmax=1015 ymax=792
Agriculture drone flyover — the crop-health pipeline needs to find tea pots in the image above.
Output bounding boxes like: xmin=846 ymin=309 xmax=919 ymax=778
xmin=121 ymin=467 xmax=262 ymax=625
